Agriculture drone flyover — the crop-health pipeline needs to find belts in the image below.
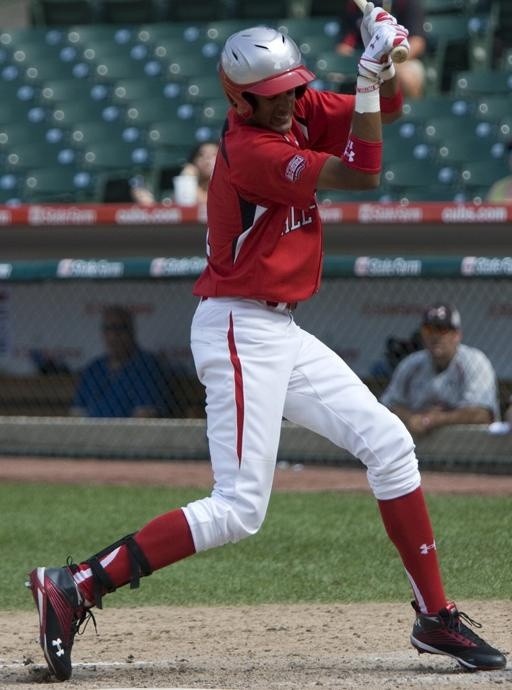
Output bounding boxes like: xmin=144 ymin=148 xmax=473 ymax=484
xmin=267 ymin=299 xmax=299 ymax=310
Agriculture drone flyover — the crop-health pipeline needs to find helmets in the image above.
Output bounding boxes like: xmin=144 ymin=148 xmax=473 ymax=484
xmin=221 ymin=26 xmax=316 ymax=119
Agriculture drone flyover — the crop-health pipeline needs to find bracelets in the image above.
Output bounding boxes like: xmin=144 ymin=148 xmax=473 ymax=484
xmin=423 ymin=414 xmax=431 ymax=431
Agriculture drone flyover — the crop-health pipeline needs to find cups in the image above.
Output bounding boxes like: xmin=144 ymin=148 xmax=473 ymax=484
xmin=172 ymin=176 xmax=198 ymax=204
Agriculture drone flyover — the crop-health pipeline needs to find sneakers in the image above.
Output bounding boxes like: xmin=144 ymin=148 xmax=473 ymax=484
xmin=29 ymin=556 xmax=97 ymax=680
xmin=409 ymin=601 xmax=506 ymax=669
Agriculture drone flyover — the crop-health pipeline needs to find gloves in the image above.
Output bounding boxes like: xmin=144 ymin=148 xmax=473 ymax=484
xmin=356 ymin=2 xmax=409 ymax=80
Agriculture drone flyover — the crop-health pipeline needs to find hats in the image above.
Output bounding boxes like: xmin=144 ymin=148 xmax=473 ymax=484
xmin=422 ymin=305 xmax=462 ymax=329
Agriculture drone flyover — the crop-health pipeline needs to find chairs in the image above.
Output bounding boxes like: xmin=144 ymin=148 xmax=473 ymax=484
xmin=1 ymin=14 xmax=512 ymax=204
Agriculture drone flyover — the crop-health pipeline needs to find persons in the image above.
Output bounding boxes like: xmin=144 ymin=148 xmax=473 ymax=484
xmin=69 ymin=305 xmax=186 ymax=418
xmin=337 ymin=0 xmax=432 ymax=98
xmin=174 ymin=141 xmax=220 ymax=207
xmin=488 ymin=174 xmax=511 ymax=205
xmin=31 ymin=1 xmax=505 ymax=682
xmin=380 ymin=301 xmax=502 ymax=433
